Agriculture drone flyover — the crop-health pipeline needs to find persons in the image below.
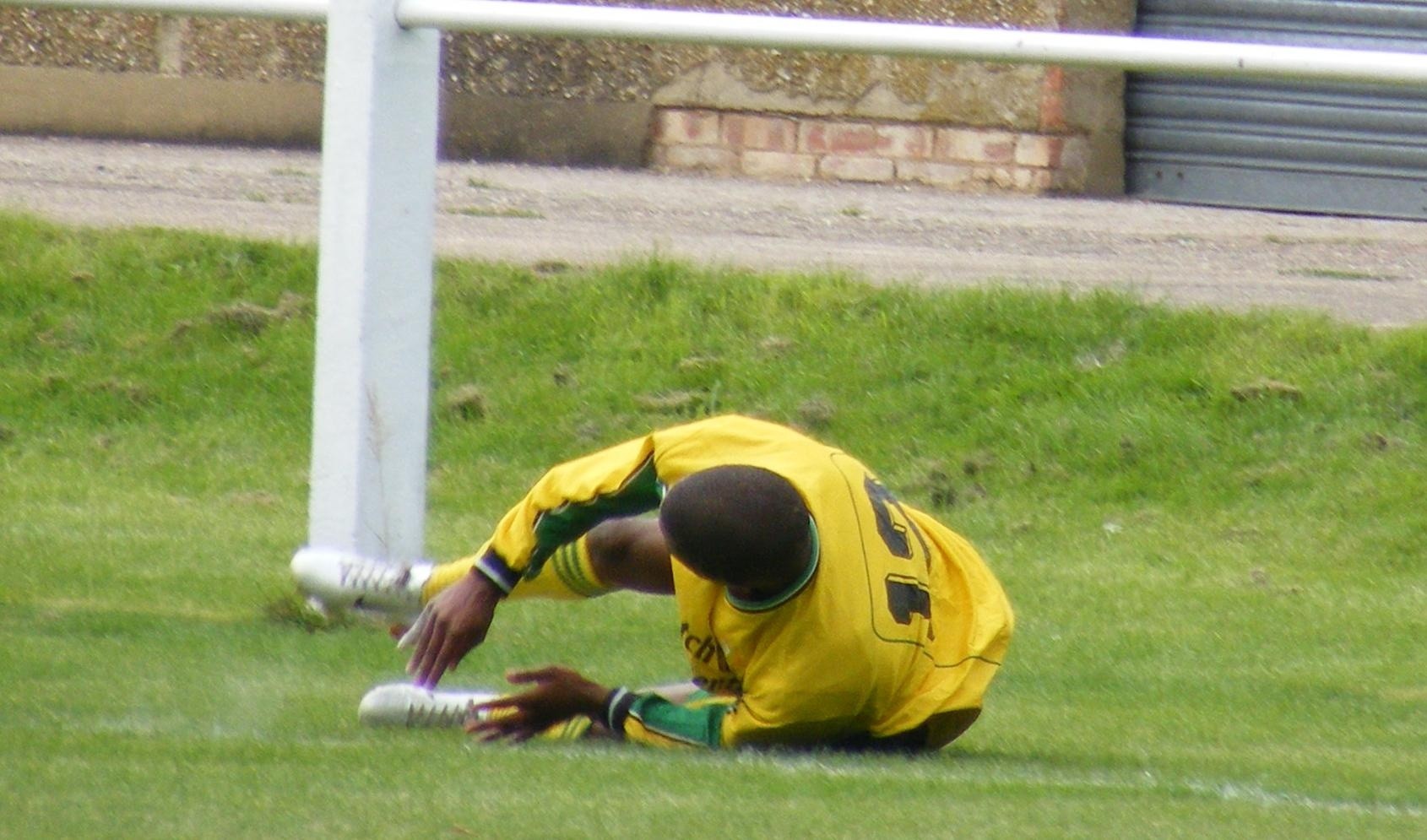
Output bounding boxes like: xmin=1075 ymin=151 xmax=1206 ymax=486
xmin=287 ymin=416 xmax=1014 ymax=752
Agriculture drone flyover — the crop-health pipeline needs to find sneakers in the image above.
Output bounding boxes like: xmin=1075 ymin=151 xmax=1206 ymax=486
xmin=291 ymin=548 xmax=433 ymax=617
xmin=357 ymin=684 xmax=495 ymax=729
